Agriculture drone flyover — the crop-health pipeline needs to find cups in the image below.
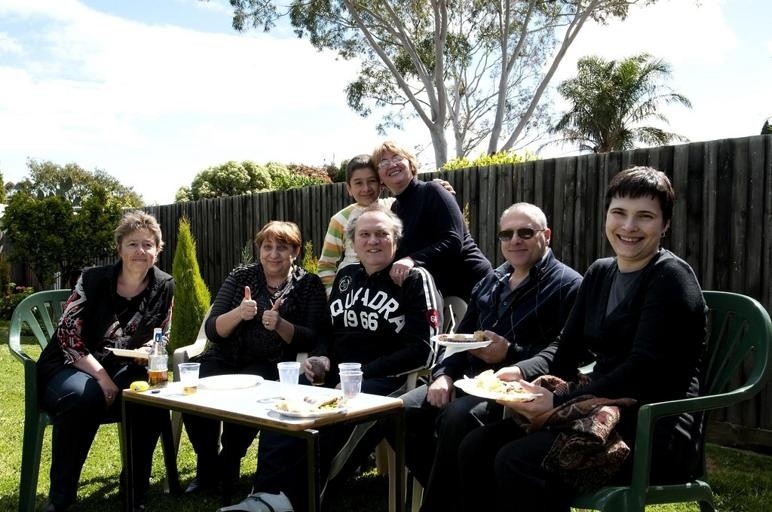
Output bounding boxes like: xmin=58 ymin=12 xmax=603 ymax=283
xmin=337 ymin=362 xmax=362 ymax=371
xmin=177 ymin=361 xmax=202 ymax=394
xmin=276 ymin=361 xmax=301 ymax=395
xmin=339 ymin=371 xmax=364 ymax=403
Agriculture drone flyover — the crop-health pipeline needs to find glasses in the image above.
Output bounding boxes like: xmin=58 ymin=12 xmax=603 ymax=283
xmin=377 ymin=155 xmax=404 ymax=169
xmin=497 ymin=228 xmax=545 ymax=241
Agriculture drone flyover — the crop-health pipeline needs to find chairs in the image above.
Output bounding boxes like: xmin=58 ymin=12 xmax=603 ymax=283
xmin=7 ymin=288 xmax=183 ymax=511
xmin=559 ymin=285 xmax=772 ymax=512
xmin=169 ymin=291 xmax=225 ymax=497
xmin=330 ymin=288 xmax=473 ymax=512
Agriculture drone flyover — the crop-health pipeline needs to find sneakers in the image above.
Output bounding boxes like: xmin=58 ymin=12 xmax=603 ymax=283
xmin=216 ymin=486 xmax=294 ymax=512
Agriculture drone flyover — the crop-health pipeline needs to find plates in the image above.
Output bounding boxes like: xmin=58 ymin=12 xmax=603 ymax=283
xmin=451 ymin=377 xmax=535 ymax=403
xmin=104 ymin=347 xmax=151 ymax=359
xmin=270 ymin=399 xmax=344 ymax=418
xmin=430 ymin=332 xmax=493 ymax=348
xmin=201 ymin=372 xmax=265 ymax=391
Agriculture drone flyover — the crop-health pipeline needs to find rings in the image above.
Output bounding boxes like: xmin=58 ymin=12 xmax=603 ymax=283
xmin=107 ymin=393 xmax=111 ymax=401
xmin=266 ymin=320 xmax=269 ymax=327
xmin=395 ymin=269 xmax=400 ymax=273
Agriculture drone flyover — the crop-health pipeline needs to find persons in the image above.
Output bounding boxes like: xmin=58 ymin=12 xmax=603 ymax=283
xmin=370 ymin=146 xmax=492 ymax=308
xmin=377 ymin=203 xmax=584 ymax=511
xmin=181 ymin=221 xmax=333 ymax=496
xmin=420 ymin=165 xmax=710 ymax=512
xmin=35 ymin=209 xmax=174 ymax=512
xmin=216 ymin=209 xmax=441 ymax=511
xmin=320 ymin=155 xmax=455 ymax=299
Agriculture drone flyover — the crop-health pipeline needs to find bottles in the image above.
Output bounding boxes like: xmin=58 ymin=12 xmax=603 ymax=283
xmin=148 ymin=326 xmax=169 ymax=387
